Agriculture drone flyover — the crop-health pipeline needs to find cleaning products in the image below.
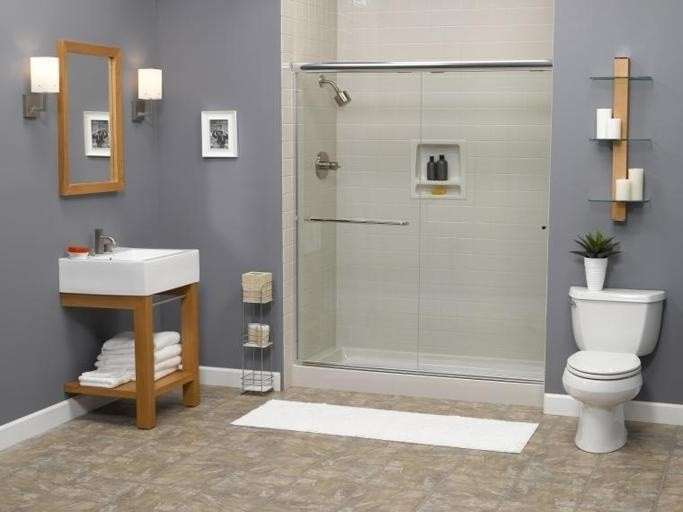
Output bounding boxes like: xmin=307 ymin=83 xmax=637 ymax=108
xmin=437 ymin=154 xmax=448 ymax=179
xmin=428 ymin=154 xmax=436 ymax=177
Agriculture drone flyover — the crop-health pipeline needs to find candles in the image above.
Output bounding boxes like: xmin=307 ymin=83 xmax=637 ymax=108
xmin=607 ymin=118 xmax=621 ymax=140
xmin=613 ymin=177 xmax=630 ymax=202
xmin=595 ymin=107 xmax=612 ymax=139
xmin=627 ymin=167 xmax=643 ymax=201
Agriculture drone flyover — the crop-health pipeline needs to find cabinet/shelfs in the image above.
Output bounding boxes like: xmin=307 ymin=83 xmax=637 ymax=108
xmin=58 ymin=282 xmax=203 ymax=432
xmin=239 ymin=278 xmax=275 ymax=399
xmin=585 ymin=76 xmax=655 ymax=205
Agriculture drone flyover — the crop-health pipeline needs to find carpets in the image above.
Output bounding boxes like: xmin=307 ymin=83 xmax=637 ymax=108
xmin=230 ymin=398 xmax=542 ymax=455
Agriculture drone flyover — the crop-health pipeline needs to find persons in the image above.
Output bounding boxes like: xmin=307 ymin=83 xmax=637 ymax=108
xmin=212 ymin=125 xmax=228 ymax=148
xmin=92 ymin=124 xmax=108 ymax=146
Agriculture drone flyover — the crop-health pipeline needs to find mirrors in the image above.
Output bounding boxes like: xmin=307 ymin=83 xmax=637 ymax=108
xmin=55 ymin=39 xmax=125 ymax=199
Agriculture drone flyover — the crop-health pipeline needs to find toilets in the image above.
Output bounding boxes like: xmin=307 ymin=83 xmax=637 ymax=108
xmin=561 ymin=287 xmax=667 ymax=456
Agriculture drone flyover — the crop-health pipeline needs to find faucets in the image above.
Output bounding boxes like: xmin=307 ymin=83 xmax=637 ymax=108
xmin=102 ymin=233 xmax=118 ymax=245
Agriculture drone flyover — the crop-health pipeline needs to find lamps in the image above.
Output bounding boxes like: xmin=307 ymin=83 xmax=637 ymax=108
xmin=22 ymin=56 xmax=60 ymax=119
xmin=131 ymin=67 xmax=165 ymax=123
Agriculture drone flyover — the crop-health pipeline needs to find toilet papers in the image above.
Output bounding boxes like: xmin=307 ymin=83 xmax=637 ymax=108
xmin=628 ymin=167 xmax=645 ymax=201
xmin=609 ymin=119 xmax=622 ymax=140
xmin=596 ymin=109 xmax=611 ymax=138
xmin=615 ymin=178 xmax=629 ymax=202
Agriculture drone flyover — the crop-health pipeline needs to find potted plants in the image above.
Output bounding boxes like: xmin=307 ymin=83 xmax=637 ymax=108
xmin=568 ymin=232 xmax=624 ymax=291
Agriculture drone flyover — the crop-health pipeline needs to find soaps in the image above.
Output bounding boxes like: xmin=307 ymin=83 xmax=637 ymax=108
xmin=67 ymin=244 xmax=90 ymax=259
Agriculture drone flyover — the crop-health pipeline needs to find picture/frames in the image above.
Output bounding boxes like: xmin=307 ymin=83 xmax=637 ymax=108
xmin=80 ymin=109 xmax=111 ymax=160
xmin=200 ymin=109 xmax=238 ymax=160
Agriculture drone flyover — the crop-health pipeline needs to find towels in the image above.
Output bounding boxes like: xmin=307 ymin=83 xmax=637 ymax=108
xmin=77 ymin=329 xmax=182 ymax=389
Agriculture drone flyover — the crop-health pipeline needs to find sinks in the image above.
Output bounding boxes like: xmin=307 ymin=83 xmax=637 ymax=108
xmin=59 ymin=248 xmax=200 ymax=299
